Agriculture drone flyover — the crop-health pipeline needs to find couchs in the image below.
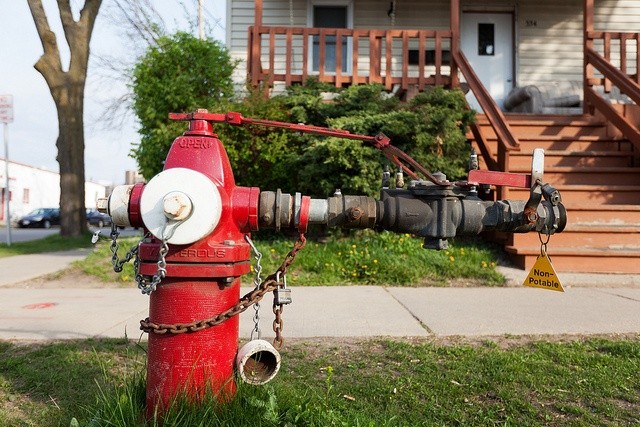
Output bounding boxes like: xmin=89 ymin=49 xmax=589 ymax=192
xmin=504 ymin=82 xmax=636 ymax=114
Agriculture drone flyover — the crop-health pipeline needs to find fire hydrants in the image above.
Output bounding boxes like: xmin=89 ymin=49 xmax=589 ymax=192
xmin=97 ymin=109 xmax=566 ymax=427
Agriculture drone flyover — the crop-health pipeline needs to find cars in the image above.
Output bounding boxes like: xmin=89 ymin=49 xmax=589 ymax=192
xmin=17 ymin=208 xmax=60 ymax=228
xmin=86 ymin=208 xmax=112 ymax=228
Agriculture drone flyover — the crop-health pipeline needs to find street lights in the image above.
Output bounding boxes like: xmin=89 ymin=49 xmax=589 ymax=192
xmin=1 ymin=94 xmax=14 ymax=247
